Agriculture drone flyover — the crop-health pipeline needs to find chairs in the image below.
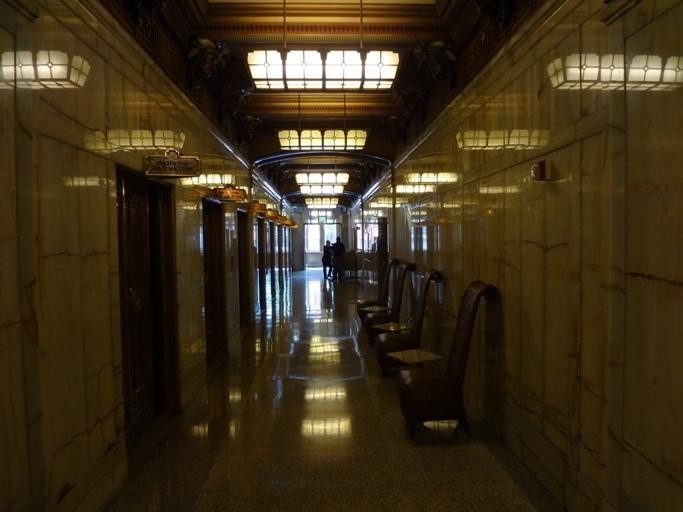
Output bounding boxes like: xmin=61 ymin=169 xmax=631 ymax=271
xmin=356 ymin=259 xmax=500 ymax=442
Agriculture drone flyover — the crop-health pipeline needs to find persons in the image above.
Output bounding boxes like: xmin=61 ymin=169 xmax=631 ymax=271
xmin=329 ymin=236 xmax=344 ymax=282
xmin=319 ymin=278 xmax=334 ymax=317
xmin=320 ymin=240 xmax=332 ymax=280
xmin=332 ymin=281 xmax=347 ymax=321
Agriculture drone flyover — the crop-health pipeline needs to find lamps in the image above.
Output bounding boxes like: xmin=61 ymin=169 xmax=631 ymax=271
xmin=246 ymin=0 xmax=398 ymax=152
xmin=455 ymin=48 xmax=681 ymax=152
xmin=2 ymin=46 xmax=187 ymax=155
